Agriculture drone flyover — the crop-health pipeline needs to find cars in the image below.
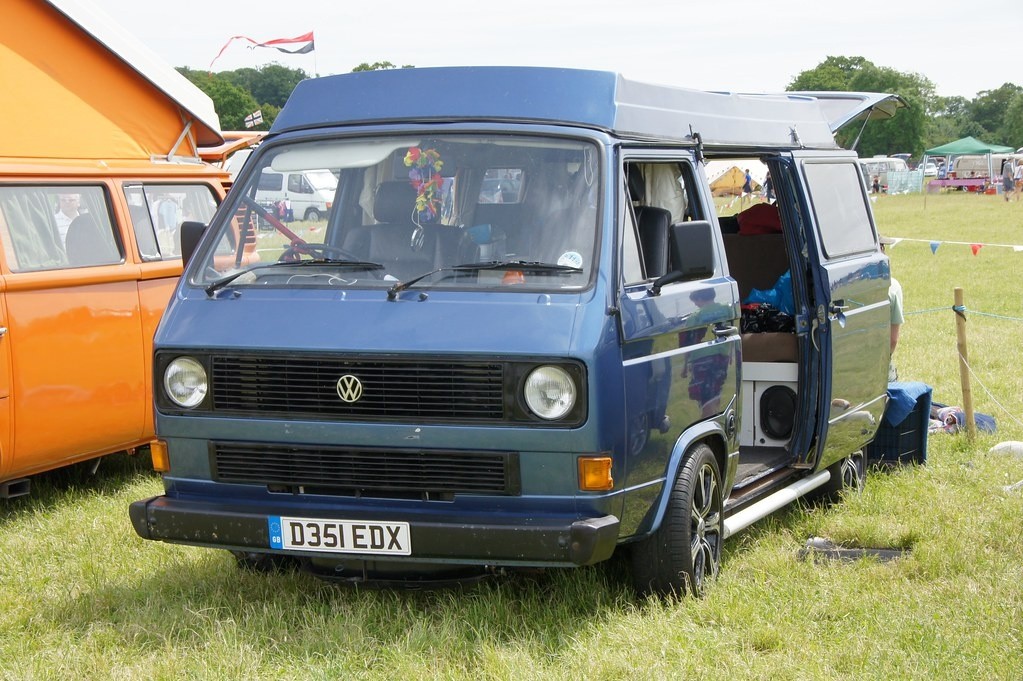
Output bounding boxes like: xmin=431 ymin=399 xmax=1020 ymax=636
xmin=916 ymin=163 xmax=940 ymax=177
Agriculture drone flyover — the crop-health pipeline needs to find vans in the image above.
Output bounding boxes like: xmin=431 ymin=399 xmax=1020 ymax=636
xmin=857 ymin=158 xmax=910 ymax=187
xmin=951 ymin=154 xmax=1023 ymax=193
xmin=127 ymin=64 xmax=912 ymax=610
xmin=1 ymin=0 xmax=260 ymax=507
xmin=210 ymin=150 xmax=341 ymax=219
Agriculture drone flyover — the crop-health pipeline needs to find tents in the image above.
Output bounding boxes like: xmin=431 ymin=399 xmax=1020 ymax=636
xmin=704 ymin=160 xmax=769 ymax=198
xmin=920 ymin=136 xmax=1015 ymax=194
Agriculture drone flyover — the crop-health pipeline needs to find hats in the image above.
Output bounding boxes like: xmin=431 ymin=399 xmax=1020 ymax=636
xmin=878 ymin=233 xmax=896 ymax=245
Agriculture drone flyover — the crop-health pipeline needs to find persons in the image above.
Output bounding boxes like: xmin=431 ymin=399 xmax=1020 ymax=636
xmin=877 ymin=232 xmax=905 ymax=382
xmin=680 ymin=289 xmax=735 ymax=421
xmin=970 ymin=171 xmax=986 ymax=192
xmin=741 ymin=168 xmax=753 ymax=206
xmin=55 ymin=194 xmax=80 ymax=252
xmin=762 ymin=171 xmax=775 ymax=203
xmin=1002 ymin=156 xmax=1023 ymax=202
xmin=872 ymin=175 xmax=880 ymax=193
xmin=271 ymin=200 xmax=287 ymax=232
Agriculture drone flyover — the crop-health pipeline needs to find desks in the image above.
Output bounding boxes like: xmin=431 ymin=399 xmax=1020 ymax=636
xmin=927 ymin=177 xmax=996 ymax=194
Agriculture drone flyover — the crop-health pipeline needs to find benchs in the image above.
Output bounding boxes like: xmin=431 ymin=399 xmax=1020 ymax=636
xmin=722 ymin=233 xmax=799 ymax=362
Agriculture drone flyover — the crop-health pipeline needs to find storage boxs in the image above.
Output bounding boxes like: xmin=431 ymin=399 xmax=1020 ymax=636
xmin=985 ymin=188 xmax=996 ymax=195
xmin=867 ymin=382 xmax=933 ymax=470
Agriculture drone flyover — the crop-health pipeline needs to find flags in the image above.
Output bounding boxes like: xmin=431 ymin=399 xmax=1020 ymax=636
xmin=244 ymin=110 xmax=263 ymax=128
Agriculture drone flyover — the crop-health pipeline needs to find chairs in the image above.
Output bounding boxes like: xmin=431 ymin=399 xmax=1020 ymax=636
xmin=66 ymin=214 xmax=117 ymax=266
xmin=337 ymin=181 xmax=458 ymax=285
xmin=554 ymin=163 xmax=670 ymax=286
xmin=129 ymin=205 xmax=158 ymax=256
